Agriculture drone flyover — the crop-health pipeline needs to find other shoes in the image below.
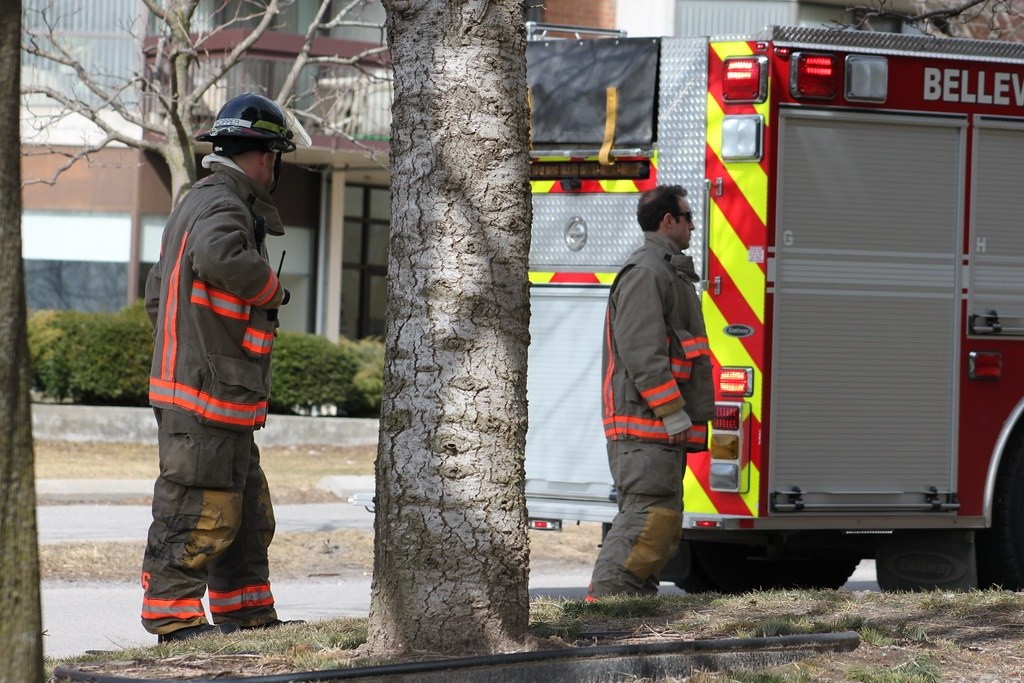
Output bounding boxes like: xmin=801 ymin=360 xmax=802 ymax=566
xmin=220 ymin=619 xmax=305 ymax=633
xmin=158 ymin=622 xmax=239 ymax=645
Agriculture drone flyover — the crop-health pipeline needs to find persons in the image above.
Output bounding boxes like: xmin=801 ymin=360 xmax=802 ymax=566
xmin=586 ymin=185 xmax=716 ymax=602
xmin=140 ymin=93 xmax=304 ymax=645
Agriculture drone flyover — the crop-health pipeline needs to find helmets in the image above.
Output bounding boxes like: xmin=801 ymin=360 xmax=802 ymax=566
xmin=194 ymin=92 xmax=298 ymax=151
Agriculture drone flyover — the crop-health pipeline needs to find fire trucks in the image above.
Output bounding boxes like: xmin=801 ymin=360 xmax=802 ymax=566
xmin=524 ymin=23 xmax=1024 ymax=591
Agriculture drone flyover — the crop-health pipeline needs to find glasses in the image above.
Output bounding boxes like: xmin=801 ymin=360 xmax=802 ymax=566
xmin=672 ymin=212 xmax=692 ymax=223
